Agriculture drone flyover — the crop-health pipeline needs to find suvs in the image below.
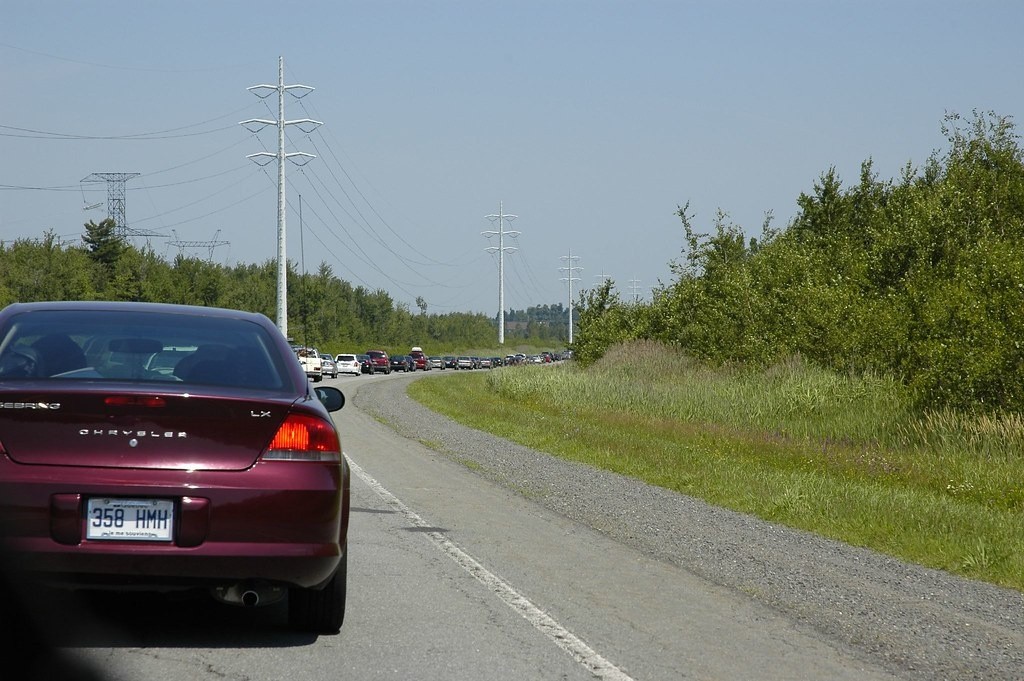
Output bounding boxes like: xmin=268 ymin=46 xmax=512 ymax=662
xmin=409 ymin=346 xmax=426 ymax=369
xmin=365 ymin=350 xmax=391 ymax=374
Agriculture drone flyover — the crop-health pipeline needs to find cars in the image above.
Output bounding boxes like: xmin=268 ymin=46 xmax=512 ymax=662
xmin=335 ymin=354 xmax=362 ymax=376
xmin=145 ymin=346 xmax=198 ymax=383
xmin=0 ymin=301 xmax=350 ymax=634
xmin=291 ymin=345 xmax=338 ymax=382
xmin=357 ymin=354 xmax=374 ymax=375
xmin=389 ymin=355 xmax=416 ymax=372
xmin=424 ymin=350 xmax=573 ymax=371
